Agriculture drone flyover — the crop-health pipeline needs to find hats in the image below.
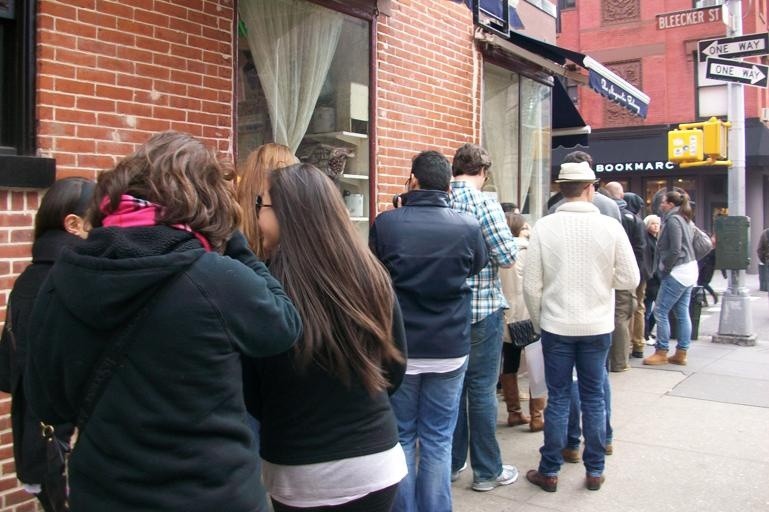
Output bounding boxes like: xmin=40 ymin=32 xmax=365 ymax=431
xmin=622 ymin=191 xmax=646 ymax=215
xmin=553 ymin=160 xmax=601 ymax=185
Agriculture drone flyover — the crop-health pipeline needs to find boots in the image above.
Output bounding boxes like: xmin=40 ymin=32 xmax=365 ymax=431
xmin=498 ymin=371 xmax=531 ymax=428
xmin=667 ymin=347 xmax=688 ymax=366
xmin=701 ymin=291 xmax=720 ymax=308
xmin=642 ymin=349 xmax=669 ymax=366
xmin=528 ymin=382 xmax=547 ymax=433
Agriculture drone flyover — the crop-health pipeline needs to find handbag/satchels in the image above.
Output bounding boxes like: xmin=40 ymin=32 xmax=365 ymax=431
xmin=505 ymin=315 xmax=542 ymax=350
xmin=37 ymin=422 xmax=75 ymax=512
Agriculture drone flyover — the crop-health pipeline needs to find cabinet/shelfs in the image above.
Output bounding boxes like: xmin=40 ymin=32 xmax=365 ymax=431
xmin=296 ymin=128 xmax=369 ymax=223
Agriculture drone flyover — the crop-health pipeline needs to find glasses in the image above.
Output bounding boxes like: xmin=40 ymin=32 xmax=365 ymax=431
xmin=253 ymin=194 xmax=275 ymax=221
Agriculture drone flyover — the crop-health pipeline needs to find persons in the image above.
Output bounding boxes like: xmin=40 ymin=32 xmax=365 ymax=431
xmin=757 ymin=227 xmax=769 ymax=268
xmin=711 ymin=234 xmax=727 ymax=279
xmin=369 ymin=150 xmax=489 ymax=512
xmin=241 ymin=162 xmax=414 ymax=512
xmin=527 ymin=161 xmax=644 ymax=492
xmin=235 ymin=141 xmax=298 ymax=258
xmin=448 ymin=141 xmax=519 ymax=493
xmin=0 ymin=177 xmax=94 ymax=511
xmin=550 ymin=149 xmax=624 ymax=222
xmin=26 ymin=131 xmax=302 ymax=511
xmin=498 ymin=181 xmax=716 ymax=430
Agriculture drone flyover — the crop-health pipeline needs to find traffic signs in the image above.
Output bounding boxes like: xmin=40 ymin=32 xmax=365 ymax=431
xmin=694 ymin=34 xmax=768 ymax=61
xmin=707 ymin=56 xmax=768 ymax=90
xmin=654 ymin=7 xmax=722 ymax=30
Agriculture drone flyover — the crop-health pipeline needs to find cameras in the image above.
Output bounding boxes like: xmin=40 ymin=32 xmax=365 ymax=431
xmin=392 ymin=193 xmax=407 ymax=208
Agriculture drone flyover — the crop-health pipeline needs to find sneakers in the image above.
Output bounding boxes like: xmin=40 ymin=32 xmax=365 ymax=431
xmin=560 ymin=446 xmax=581 ymax=464
xmin=610 ymin=363 xmax=633 ymax=373
xmin=603 ymin=441 xmax=614 ymax=457
xmin=469 ymin=463 xmax=520 ymax=493
xmin=450 ymin=461 xmax=469 ymax=485
xmin=631 ymin=332 xmax=659 ymax=359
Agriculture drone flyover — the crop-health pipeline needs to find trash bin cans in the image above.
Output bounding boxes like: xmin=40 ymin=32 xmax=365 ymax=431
xmin=759 ymin=263 xmax=769 ymax=291
xmin=669 ymin=286 xmax=704 ymax=340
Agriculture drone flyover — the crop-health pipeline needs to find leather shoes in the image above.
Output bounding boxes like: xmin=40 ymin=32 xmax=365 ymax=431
xmin=585 ymin=470 xmax=606 ymax=491
xmin=525 ymin=469 xmax=559 ymax=494
